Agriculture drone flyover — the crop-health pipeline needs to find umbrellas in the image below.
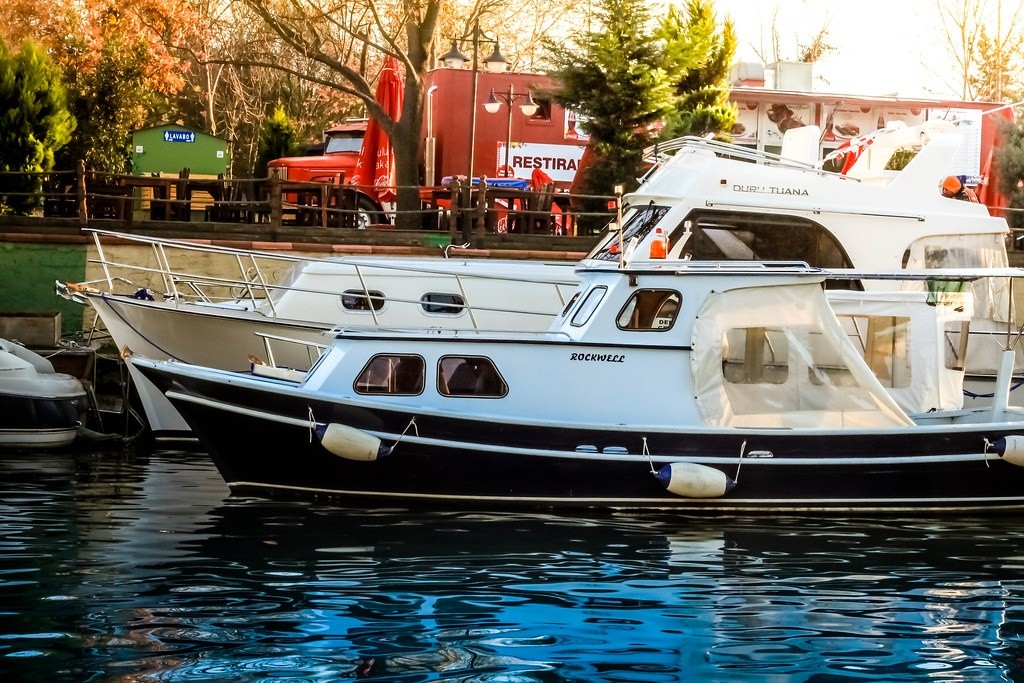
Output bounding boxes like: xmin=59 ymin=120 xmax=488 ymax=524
xmin=975 ymin=101 xmax=1017 ymax=220
xmin=350 ymin=51 xmax=404 ymax=226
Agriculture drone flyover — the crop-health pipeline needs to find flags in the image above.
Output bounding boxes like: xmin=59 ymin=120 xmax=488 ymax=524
xmin=829 ymin=125 xmax=893 ymax=185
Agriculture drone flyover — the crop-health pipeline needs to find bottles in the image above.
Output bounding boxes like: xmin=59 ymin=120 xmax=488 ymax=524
xmin=649 ymin=228 xmax=666 ymax=259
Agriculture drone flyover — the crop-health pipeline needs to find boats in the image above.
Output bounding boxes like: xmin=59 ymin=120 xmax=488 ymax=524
xmin=128 ymin=255 xmax=1024 ymax=517
xmin=0 ymin=337 xmax=87 ymax=448
xmin=49 ymin=117 xmax=1013 ymax=447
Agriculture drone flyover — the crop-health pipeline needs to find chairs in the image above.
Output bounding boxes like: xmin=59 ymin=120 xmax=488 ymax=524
xmin=780 ymin=126 xmax=820 ymax=174
xmin=47 ymin=160 xmax=621 ymax=233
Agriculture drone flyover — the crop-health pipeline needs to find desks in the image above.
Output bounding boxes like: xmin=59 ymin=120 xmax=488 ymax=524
xmin=433 ymin=187 xmax=537 ymax=234
xmin=747 ymin=288 xmax=975 ymax=415
xmin=258 ymin=182 xmax=328 ymax=227
xmin=119 ymin=172 xmax=222 ymax=225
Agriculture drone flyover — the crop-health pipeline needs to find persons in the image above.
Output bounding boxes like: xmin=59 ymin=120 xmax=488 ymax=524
xmin=942 ymin=176 xmax=979 ymax=203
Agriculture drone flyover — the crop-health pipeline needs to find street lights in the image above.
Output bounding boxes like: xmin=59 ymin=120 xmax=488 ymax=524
xmin=438 ymin=18 xmax=513 ymax=243
xmin=482 ymin=84 xmax=541 ymax=177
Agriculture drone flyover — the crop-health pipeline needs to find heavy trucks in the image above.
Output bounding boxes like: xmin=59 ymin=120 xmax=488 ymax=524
xmin=267 ymin=71 xmax=1013 ymax=229
